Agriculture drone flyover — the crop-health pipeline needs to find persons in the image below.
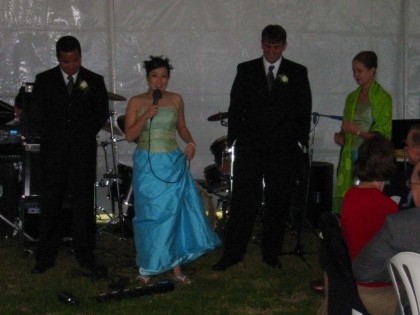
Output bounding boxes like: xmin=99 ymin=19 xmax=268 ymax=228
xmin=212 ymin=24 xmax=313 ymax=269
xmin=310 ymin=126 xmax=420 ymax=315
xmin=11 ymin=36 xmax=111 ymax=282
xmin=124 ymin=57 xmax=222 ymax=287
xmin=332 ymin=52 xmax=393 ymax=224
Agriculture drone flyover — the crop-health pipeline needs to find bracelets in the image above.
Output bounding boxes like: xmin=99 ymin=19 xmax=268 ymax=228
xmin=357 ymin=128 xmax=360 ymax=136
xmin=189 ymin=142 xmax=196 ymax=148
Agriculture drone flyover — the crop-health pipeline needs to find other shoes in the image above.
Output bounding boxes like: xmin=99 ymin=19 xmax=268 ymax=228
xmin=310 ymin=279 xmax=325 ymax=292
xmin=261 ymin=242 xmax=279 ymax=266
xmin=79 ymin=258 xmax=105 ymax=277
xmin=31 ymin=256 xmax=55 ymax=273
xmin=212 ymin=251 xmax=244 ymax=270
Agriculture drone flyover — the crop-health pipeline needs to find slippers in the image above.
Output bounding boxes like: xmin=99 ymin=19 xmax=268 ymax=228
xmin=136 ymin=274 xmax=154 ymax=287
xmin=172 ymin=274 xmax=191 ymax=284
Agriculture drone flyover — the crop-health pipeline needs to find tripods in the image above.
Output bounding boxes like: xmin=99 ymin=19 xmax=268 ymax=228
xmin=278 ymin=115 xmax=343 ymax=267
xmin=96 ymin=135 xmax=133 ymax=240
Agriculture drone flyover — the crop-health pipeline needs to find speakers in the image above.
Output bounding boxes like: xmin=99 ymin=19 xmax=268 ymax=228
xmin=302 ymin=160 xmax=333 ymax=229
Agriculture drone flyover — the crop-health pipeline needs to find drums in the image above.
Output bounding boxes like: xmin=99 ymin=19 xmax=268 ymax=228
xmin=203 ymin=133 xmax=266 ymax=197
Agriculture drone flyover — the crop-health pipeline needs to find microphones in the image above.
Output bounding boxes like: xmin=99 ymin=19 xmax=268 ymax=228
xmin=151 ymin=89 xmax=162 ymax=120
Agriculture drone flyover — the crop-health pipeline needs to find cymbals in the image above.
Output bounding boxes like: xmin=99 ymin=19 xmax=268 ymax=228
xmin=105 ymin=91 xmax=128 ymax=101
xmin=117 ymin=114 xmax=140 ymax=143
xmin=207 ymin=111 xmax=229 ymax=122
xmin=101 ymin=122 xmax=124 ymax=136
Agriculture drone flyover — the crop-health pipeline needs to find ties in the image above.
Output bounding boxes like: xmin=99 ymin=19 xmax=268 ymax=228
xmin=67 ymin=74 xmax=74 ymax=96
xmin=266 ymin=66 xmax=275 ymax=92
